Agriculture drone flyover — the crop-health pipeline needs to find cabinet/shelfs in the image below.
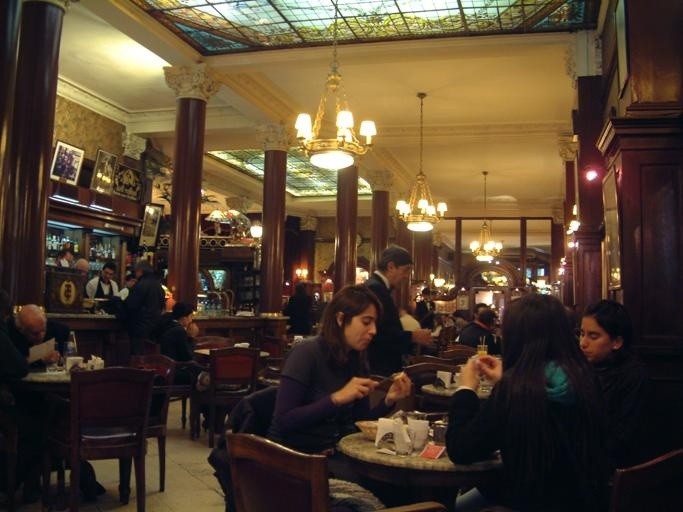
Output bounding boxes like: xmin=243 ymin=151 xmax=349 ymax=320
xmin=236 ymin=269 xmax=261 ymax=313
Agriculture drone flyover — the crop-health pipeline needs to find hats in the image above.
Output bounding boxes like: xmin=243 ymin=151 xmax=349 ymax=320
xmin=172 ymin=302 xmax=193 ymax=316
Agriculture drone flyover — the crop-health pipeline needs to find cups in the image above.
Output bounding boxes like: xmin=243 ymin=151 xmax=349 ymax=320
xmin=479 ymin=380 xmax=491 ymax=392
xmin=374 ymin=416 xmax=394 ymax=449
xmin=435 ymin=370 xmax=452 ymax=388
xmin=293 ymin=335 xmax=303 ymax=344
xmin=476 ymin=344 xmax=486 ymax=356
xmin=65 ymin=356 xmax=84 ymax=371
xmin=407 ymin=419 xmax=430 ymax=450
xmin=88 ymin=260 xmax=104 ymax=270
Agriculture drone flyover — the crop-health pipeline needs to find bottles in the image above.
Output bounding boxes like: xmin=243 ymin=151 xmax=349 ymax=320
xmin=95 ymin=241 xmax=112 ymax=259
xmin=198 ymin=299 xmax=222 ymax=310
xmin=45 ymin=232 xmax=79 ymax=254
xmin=239 ymin=301 xmax=254 ymax=312
xmin=125 ymin=246 xmax=153 ymax=267
xmin=66 ymin=331 xmax=77 ymax=355
xmin=229 ymin=305 xmax=232 ymax=316
xmin=392 ymin=417 xmax=415 ymax=455
xmin=232 ymin=304 xmax=237 ymax=315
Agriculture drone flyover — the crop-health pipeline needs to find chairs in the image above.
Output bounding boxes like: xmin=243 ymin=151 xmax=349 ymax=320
xmin=189 ymin=330 xmax=319 ymax=368
xmin=334 ymin=325 xmax=683 ymax=512
xmin=199 ymin=346 xmax=259 ymax=448
xmin=225 ymin=433 xmax=444 ymax=512
xmin=134 ymin=340 xmax=200 ymax=437
xmin=128 ymin=355 xmax=176 ymax=492
xmin=2 ymin=370 xmax=65 ymax=512
xmin=45 ymin=370 xmax=155 ymax=512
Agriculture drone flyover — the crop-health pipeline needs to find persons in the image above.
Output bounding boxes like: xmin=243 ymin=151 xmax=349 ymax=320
xmin=283 ymin=284 xmax=312 ymax=336
xmin=580 ymin=301 xmax=649 ymax=468
xmin=446 ymin=294 xmax=603 ymax=512
xmin=0 ymin=290 xmax=106 ymax=511
xmin=162 ymin=304 xmax=221 ymax=427
xmin=270 ymin=285 xmax=412 ymax=506
xmin=53 ymin=249 xmax=137 ymax=300
xmin=399 ymin=288 xmax=436 ymax=331
xmin=364 ymin=246 xmax=432 ymax=376
xmin=116 ymin=260 xmax=164 ymax=352
xmin=452 ymin=303 xmax=502 ymax=355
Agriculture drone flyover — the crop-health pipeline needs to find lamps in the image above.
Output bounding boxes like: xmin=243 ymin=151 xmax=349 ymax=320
xmin=554 ymin=205 xmax=580 ymax=293
xmin=470 ymin=171 xmax=504 ymax=264
xmin=205 ymin=207 xmax=231 ymax=236
xmin=395 ymin=93 xmax=448 ymax=232
xmin=294 ymin=0 xmax=377 ymax=171
xmin=250 ymin=220 xmax=262 ymax=269
xmin=586 ymin=170 xmax=597 ymax=181
xmin=89 ymin=193 xmax=114 ymax=212
xmin=52 ymin=181 xmax=80 ymax=203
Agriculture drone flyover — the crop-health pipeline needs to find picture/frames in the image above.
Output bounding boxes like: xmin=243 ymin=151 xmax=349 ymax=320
xmin=138 ymin=202 xmax=164 ymax=248
xmin=89 ymin=148 xmax=119 ymax=196
xmin=50 ymin=139 xmax=85 ymax=187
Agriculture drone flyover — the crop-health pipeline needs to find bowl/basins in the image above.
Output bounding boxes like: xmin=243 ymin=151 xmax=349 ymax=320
xmin=355 ymin=420 xmax=376 ymax=441
